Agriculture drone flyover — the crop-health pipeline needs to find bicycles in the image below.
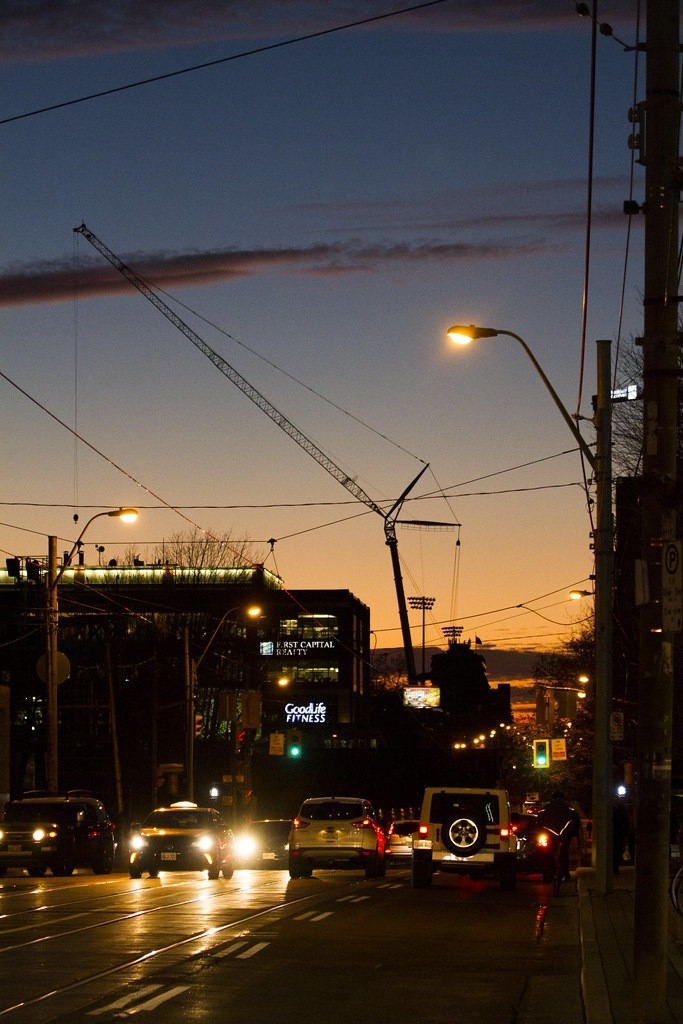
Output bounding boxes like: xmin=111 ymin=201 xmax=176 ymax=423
xmin=670 ymin=865 xmax=683 ymax=916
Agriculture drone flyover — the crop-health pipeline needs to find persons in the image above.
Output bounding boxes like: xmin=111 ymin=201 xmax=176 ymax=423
xmin=539 ymin=790 xmax=585 ymax=883
xmin=612 ymin=804 xmax=635 ymax=874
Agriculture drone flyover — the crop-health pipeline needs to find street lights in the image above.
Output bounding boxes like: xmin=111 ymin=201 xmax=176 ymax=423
xmin=48 ymin=507 xmax=142 ymax=795
xmin=189 ymin=602 xmax=261 ymax=801
xmin=446 ymin=321 xmax=615 ymax=900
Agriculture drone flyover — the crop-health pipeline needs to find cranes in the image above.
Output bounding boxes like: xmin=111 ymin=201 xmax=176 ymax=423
xmin=71 ymin=219 xmax=463 ymax=692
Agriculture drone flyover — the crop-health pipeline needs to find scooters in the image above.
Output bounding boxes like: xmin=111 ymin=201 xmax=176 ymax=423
xmin=550 ymin=837 xmax=572 ymax=893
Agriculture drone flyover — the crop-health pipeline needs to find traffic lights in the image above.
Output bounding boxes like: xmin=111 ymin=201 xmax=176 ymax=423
xmin=286 ymin=730 xmax=303 ymax=759
xmin=533 ymin=739 xmax=550 ymax=768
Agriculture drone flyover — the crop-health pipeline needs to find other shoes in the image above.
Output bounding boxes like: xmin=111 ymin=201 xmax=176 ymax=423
xmin=563 ymin=869 xmax=570 ymax=881
xmin=543 ymin=871 xmax=553 ymax=883
xmin=613 ymin=867 xmax=620 ymax=875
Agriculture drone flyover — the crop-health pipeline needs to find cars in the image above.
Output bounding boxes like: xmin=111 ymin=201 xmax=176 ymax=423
xmin=128 ymin=801 xmax=234 ymax=883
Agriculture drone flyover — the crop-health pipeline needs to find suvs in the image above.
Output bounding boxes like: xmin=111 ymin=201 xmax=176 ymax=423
xmin=235 ymin=788 xmax=554 ymax=889
xmin=0 ymin=790 xmax=119 ymax=878
